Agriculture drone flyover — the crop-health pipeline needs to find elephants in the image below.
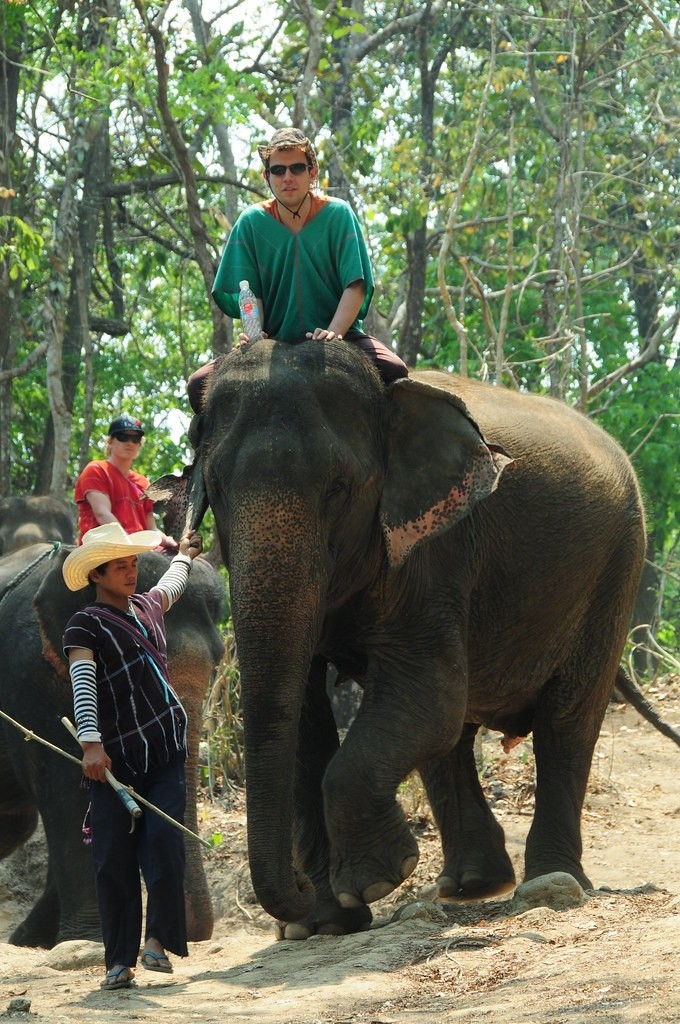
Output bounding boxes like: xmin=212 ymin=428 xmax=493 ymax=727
xmin=143 ymin=333 xmax=680 ymax=941
xmin=0 ymin=491 xmax=227 ymax=949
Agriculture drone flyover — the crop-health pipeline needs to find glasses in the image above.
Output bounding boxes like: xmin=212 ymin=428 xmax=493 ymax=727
xmin=265 ymin=162 xmax=313 ymax=176
xmin=112 ymin=431 xmax=142 ymax=444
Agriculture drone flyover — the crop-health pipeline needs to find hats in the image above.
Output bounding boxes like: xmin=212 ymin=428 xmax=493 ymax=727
xmin=258 ymin=127 xmax=317 ymax=170
xmin=108 ymin=415 xmax=145 ymax=437
xmin=62 ymin=522 xmax=162 ymax=591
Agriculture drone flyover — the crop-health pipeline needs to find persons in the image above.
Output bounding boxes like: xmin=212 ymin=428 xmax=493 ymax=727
xmin=210 ymin=127 xmax=408 ymax=379
xmin=61 ymin=418 xmax=203 ymax=991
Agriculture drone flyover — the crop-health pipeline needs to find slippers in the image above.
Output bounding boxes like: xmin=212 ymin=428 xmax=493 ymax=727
xmin=102 ymin=966 xmax=131 ymax=989
xmin=141 ymin=951 xmax=173 ymax=973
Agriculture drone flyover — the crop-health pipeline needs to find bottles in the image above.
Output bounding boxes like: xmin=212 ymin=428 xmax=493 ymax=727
xmin=237 ymin=280 xmax=262 ymax=340
